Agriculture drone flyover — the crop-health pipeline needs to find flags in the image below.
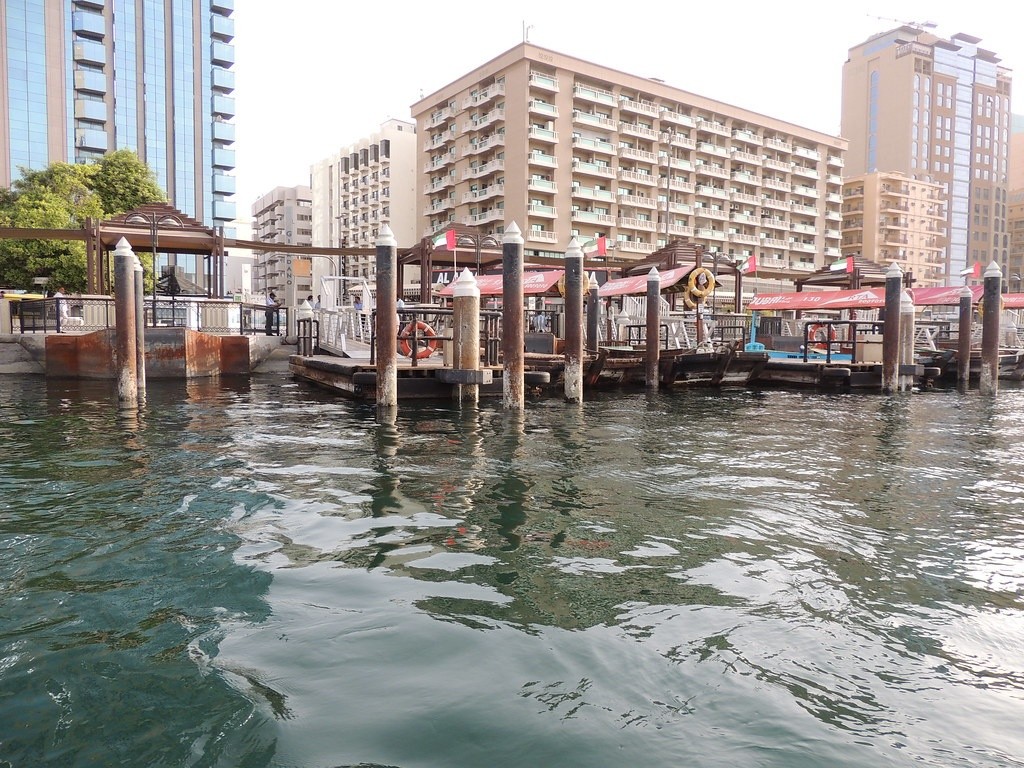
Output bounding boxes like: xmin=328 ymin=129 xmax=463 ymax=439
xmin=830 ymin=257 xmax=853 ymax=275
xmin=432 ymin=229 xmax=454 ymax=252
xmin=960 ymin=262 xmax=979 ymax=279
xmin=581 ymin=236 xmax=606 ymax=258
xmin=736 ymin=256 xmax=756 ymax=274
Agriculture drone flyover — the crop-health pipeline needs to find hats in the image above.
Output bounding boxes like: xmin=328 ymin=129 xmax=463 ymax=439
xmin=270 ymin=293 xmax=277 ymax=297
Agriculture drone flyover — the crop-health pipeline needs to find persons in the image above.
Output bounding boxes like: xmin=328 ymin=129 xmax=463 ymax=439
xmin=354 ymin=297 xmax=363 ymax=310
xmin=532 ymin=312 xmax=547 ymax=332
xmin=397 ymin=294 xmax=404 ymax=305
xmin=53 ymin=287 xmax=68 ymax=333
xmin=307 ymin=295 xmax=315 ymax=309
xmin=583 ymin=301 xmax=587 ymax=312
xmin=315 ymin=295 xmax=320 ymax=308
xmin=820 ymin=331 xmax=826 ymax=341
xmin=265 ymin=293 xmax=281 ymax=336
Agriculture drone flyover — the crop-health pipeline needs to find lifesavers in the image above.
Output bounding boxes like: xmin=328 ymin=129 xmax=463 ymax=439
xmin=687 ymin=267 xmax=716 ymax=298
xmin=684 ymin=284 xmax=708 ymax=310
xmin=978 ymin=294 xmax=1005 ymax=320
xmin=809 ymin=323 xmax=837 ymax=349
xmin=399 ymin=320 xmax=438 ymax=359
xmin=558 ymin=271 xmax=590 ymax=299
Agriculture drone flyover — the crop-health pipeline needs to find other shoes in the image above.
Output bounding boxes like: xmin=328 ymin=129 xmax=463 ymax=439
xmin=266 ymin=333 xmax=275 ymax=336
xmin=61 ymin=330 xmax=66 ymax=333
